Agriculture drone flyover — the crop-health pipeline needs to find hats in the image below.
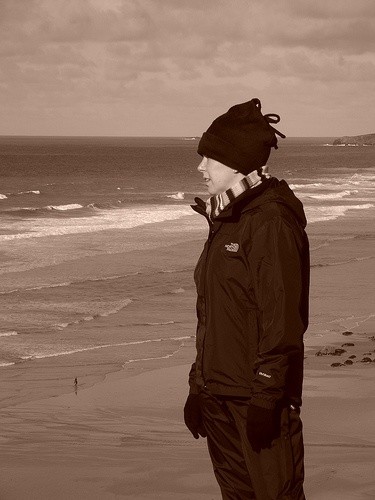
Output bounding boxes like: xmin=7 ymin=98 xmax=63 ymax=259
xmin=197 ymin=97 xmax=286 ymax=176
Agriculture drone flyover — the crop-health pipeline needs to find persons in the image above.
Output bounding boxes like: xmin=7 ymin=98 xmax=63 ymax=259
xmin=182 ymin=98 xmax=310 ymax=500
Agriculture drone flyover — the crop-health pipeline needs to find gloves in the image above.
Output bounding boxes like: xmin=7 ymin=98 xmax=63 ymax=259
xmin=246 ymin=403 xmax=282 ymax=455
xmin=184 ymin=394 xmax=208 ymax=439
